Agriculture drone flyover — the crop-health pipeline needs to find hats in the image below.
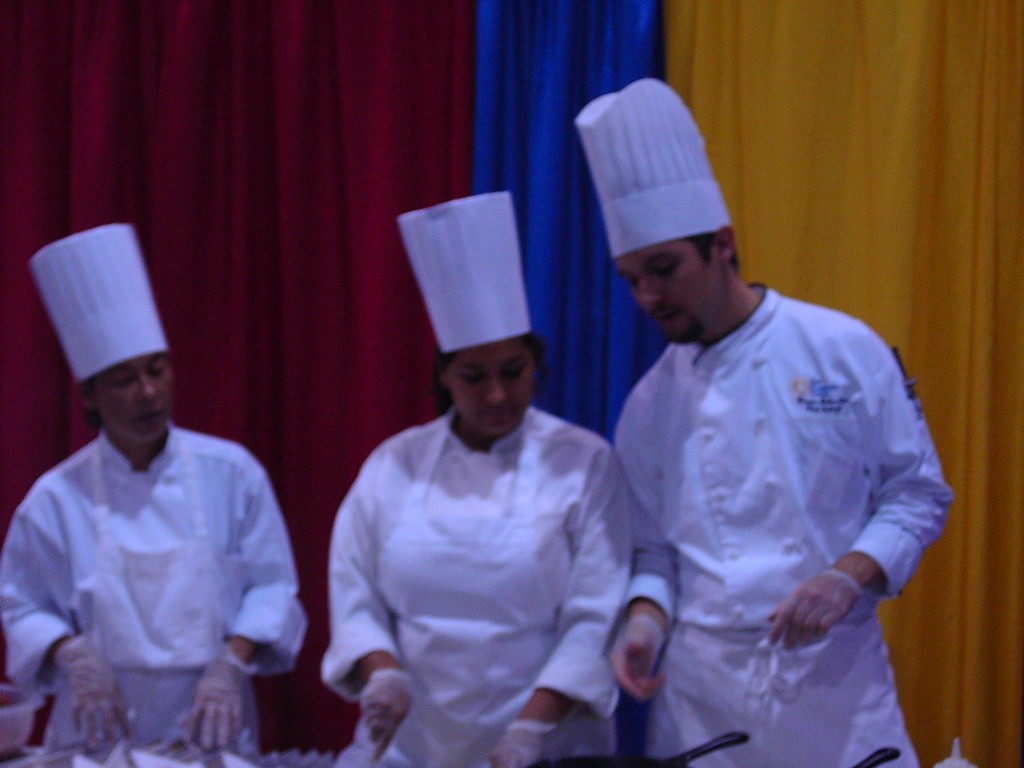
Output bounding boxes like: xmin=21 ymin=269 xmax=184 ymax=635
xmin=575 ymin=79 xmax=731 ymax=258
xmin=28 ymin=222 xmax=169 ymax=381
xmin=397 ymin=191 xmax=529 ymax=355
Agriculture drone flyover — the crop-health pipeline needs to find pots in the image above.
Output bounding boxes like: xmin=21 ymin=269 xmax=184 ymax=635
xmin=528 ymin=732 xmax=749 ymax=768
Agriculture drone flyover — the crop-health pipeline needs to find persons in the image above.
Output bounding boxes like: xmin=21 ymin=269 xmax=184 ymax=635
xmin=575 ymin=78 xmax=956 ymax=768
xmin=320 ymin=191 xmax=632 ymax=768
xmin=1 ymin=223 xmax=309 ymax=757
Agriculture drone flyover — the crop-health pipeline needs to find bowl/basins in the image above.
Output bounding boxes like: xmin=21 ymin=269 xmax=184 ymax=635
xmin=0 ymin=683 xmax=46 ymax=761
